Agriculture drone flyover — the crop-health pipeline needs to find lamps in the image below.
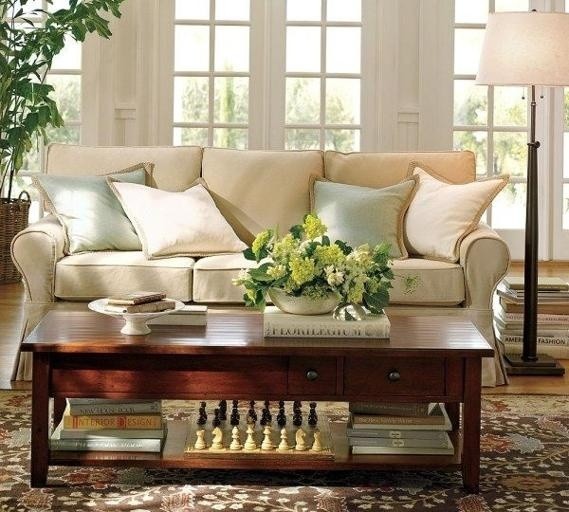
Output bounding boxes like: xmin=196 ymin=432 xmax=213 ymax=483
xmin=473 ymin=9 xmax=569 ymax=375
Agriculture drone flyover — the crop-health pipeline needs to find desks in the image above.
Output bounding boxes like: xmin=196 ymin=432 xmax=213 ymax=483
xmin=19 ymin=308 xmax=495 ymax=494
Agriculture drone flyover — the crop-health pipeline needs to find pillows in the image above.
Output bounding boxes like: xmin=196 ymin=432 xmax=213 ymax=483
xmin=44 ymin=142 xmax=203 ymax=211
xmin=106 ymin=174 xmax=250 ymax=260
xmin=31 ymin=162 xmax=152 ymax=256
xmin=201 ymin=145 xmax=324 ymax=251
xmin=324 ymin=148 xmax=475 ymax=200
xmin=404 ymin=162 xmax=508 ymax=264
xmin=309 ymin=173 xmax=421 ymax=260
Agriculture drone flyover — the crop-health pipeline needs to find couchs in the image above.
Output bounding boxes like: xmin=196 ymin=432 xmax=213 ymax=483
xmin=8 ymin=140 xmax=511 ymax=390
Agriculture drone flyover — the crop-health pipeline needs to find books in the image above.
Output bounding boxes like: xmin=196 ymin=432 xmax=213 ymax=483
xmin=104 ymin=300 xmax=175 ymax=313
xmin=345 ymin=402 xmax=455 ymax=455
xmin=263 ymin=305 xmax=391 ymax=339
xmin=108 ymin=291 xmax=166 ymax=305
xmin=49 ymin=399 xmax=170 ymax=452
xmin=145 ymin=305 xmax=207 ymax=326
xmin=494 ymin=277 xmax=569 ymax=362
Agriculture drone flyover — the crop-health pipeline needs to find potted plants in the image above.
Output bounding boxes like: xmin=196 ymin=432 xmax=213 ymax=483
xmin=0 ymin=0 xmax=122 ymax=285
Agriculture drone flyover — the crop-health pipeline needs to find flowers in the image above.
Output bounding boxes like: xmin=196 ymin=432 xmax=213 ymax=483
xmin=233 ymin=214 xmax=421 ymax=323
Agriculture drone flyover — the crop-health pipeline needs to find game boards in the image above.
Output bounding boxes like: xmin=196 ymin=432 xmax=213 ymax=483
xmin=184 ymin=413 xmax=334 ymax=461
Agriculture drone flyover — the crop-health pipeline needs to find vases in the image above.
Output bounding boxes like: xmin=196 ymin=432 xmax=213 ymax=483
xmin=267 ymin=286 xmax=340 ymax=315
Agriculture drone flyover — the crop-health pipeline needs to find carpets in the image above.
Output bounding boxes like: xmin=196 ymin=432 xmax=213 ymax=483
xmin=0 ymin=386 xmax=568 ymax=511
